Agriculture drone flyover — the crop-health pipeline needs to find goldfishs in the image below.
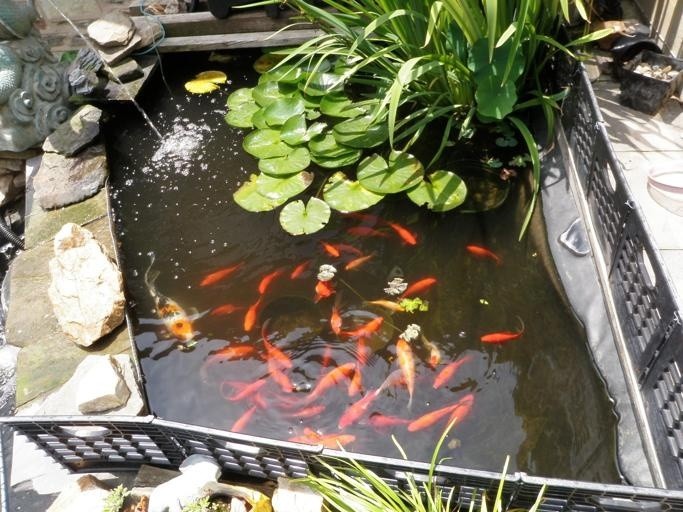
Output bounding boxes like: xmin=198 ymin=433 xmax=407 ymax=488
xmin=207 ymin=298 xmax=477 ymax=450
